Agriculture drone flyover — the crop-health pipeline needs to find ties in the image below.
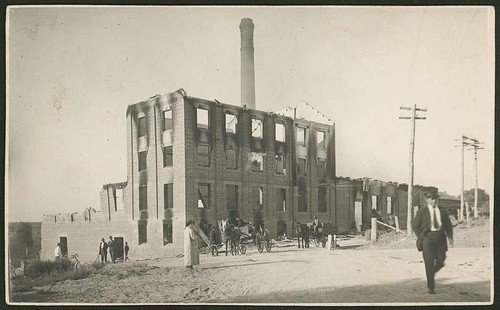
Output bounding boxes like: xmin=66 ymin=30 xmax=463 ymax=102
xmin=433 ymin=205 xmax=439 ymax=229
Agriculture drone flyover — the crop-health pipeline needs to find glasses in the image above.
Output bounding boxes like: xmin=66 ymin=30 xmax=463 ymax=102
xmin=431 ymin=197 xmax=437 ymax=200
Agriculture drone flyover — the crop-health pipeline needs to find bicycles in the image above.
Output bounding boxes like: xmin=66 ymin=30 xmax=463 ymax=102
xmin=69 ymin=253 xmax=90 ymax=274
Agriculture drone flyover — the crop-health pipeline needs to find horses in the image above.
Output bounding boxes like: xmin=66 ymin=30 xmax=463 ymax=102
xmin=219 ymin=218 xmax=240 ymax=256
xmin=296 ymin=222 xmax=310 ymax=248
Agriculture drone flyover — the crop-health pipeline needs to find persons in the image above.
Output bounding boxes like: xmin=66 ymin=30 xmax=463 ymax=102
xmin=99 ymin=238 xmax=108 ymax=262
xmin=55 ymin=243 xmax=61 ymax=261
xmin=253 ymin=205 xmax=264 ymax=233
xmin=184 ymin=220 xmax=199 ymax=267
xmin=413 ymin=186 xmax=453 ymax=294
xmin=370 ymin=208 xmax=379 ymax=229
xmin=208 ymin=224 xmax=218 ymax=255
xmin=108 ymin=236 xmax=116 ymax=263
xmin=313 ymin=217 xmax=322 ymax=235
xmin=124 ymin=242 xmax=129 ymax=261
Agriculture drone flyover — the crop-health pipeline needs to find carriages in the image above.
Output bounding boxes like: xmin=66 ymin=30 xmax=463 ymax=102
xmin=224 ymin=220 xmax=273 ymax=256
xmin=296 ymin=222 xmax=339 ymax=249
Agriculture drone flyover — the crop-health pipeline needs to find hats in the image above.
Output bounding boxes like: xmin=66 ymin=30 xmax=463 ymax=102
xmin=101 ymin=238 xmax=105 ymax=241
xmin=426 ymin=192 xmax=440 ymax=198
xmin=186 ymin=218 xmax=195 ymax=225
xmin=57 ymin=243 xmax=61 ymax=246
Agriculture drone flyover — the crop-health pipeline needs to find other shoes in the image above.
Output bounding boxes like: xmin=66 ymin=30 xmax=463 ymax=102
xmin=428 ymin=288 xmax=436 ymax=294
